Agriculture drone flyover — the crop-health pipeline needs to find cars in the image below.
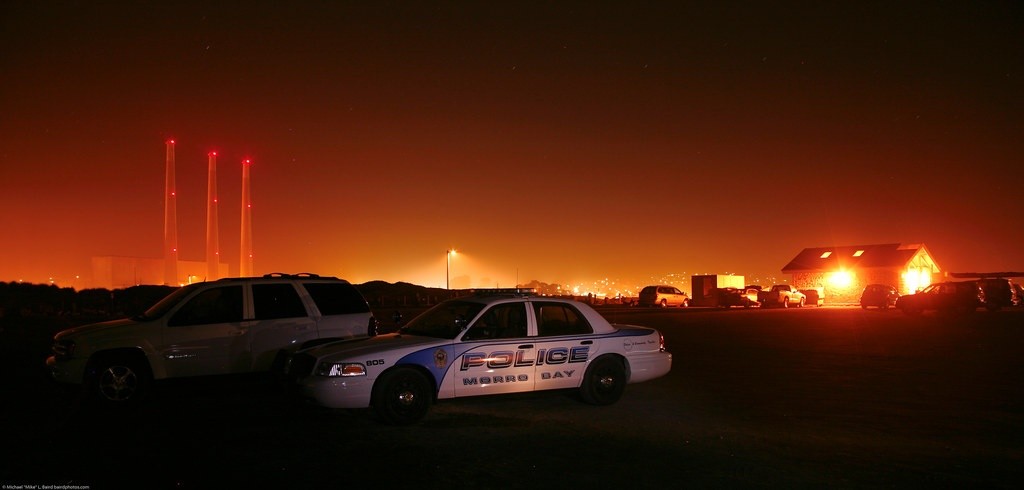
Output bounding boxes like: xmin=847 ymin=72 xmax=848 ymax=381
xmin=860 ymin=283 xmax=901 ymax=310
xmin=283 ymin=288 xmax=672 ymax=428
xmin=715 ymin=282 xmax=824 ymax=310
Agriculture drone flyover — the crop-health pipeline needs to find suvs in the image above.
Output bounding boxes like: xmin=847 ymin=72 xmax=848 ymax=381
xmin=896 ymin=281 xmax=990 ymax=314
xmin=639 ymin=285 xmax=691 ymax=310
xmin=968 ymin=277 xmax=1024 ymax=310
xmin=47 ymin=272 xmax=380 ymax=410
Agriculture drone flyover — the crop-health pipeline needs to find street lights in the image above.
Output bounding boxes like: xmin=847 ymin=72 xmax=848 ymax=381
xmin=447 ymin=249 xmax=456 ymax=289
